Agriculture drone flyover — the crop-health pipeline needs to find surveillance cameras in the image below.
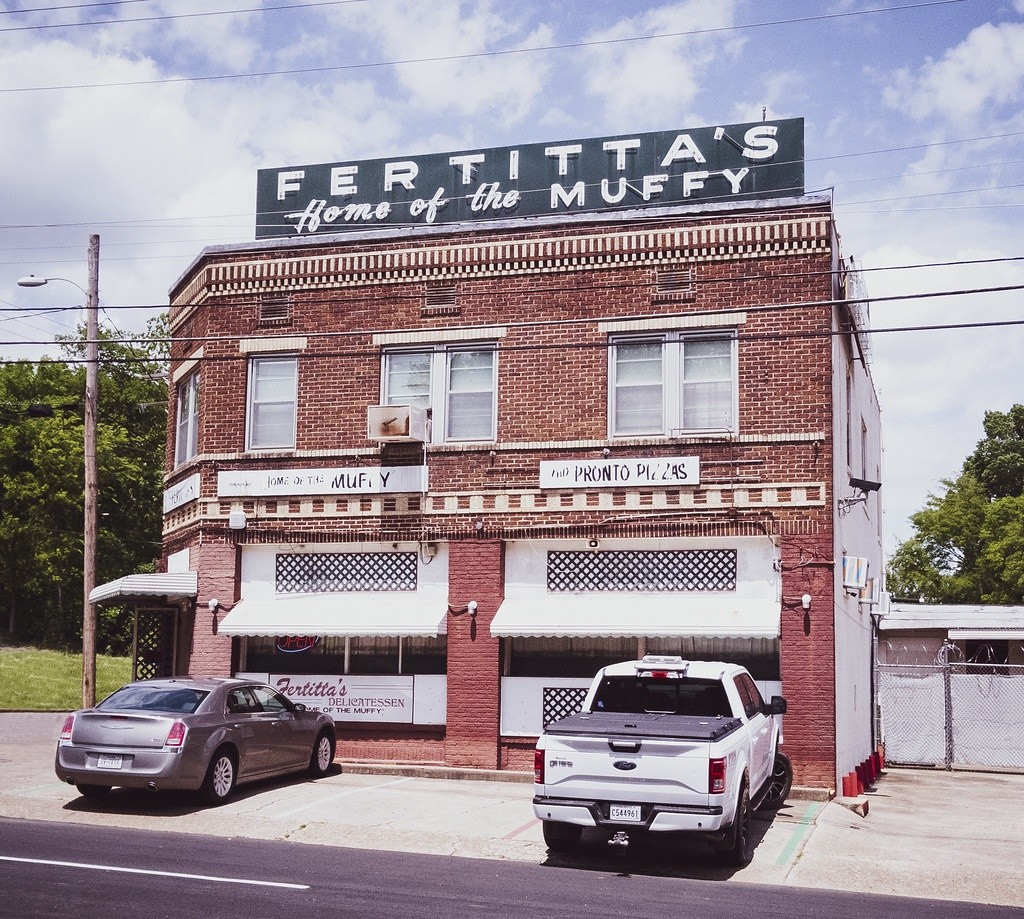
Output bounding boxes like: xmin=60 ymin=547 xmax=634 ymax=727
xmin=849 ymin=478 xmax=882 ymax=491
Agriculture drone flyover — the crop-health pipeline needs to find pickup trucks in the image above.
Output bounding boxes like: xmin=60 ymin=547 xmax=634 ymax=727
xmin=532 ymin=653 xmax=793 ymax=867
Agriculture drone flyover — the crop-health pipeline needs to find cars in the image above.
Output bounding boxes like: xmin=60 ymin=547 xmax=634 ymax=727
xmin=54 ymin=675 xmax=339 ymax=805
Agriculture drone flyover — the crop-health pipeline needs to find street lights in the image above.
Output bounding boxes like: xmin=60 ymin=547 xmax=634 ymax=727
xmin=16 ymin=270 xmax=102 ymax=709
